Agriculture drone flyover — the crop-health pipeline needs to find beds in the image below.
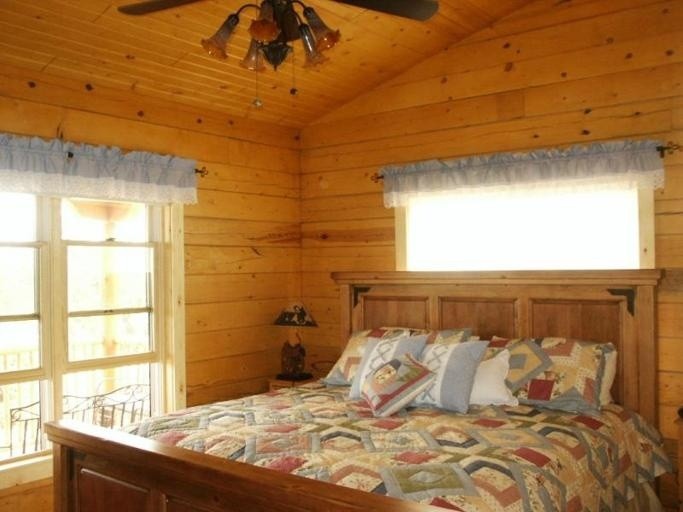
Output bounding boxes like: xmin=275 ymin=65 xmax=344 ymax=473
xmin=45 ymin=270 xmax=665 ymax=512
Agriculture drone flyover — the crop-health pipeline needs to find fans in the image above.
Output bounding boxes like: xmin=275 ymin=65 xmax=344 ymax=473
xmin=117 ymin=0 xmax=438 ymax=21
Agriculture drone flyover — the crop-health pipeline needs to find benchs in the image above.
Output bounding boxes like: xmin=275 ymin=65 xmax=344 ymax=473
xmin=0 ymin=384 xmax=149 ymax=458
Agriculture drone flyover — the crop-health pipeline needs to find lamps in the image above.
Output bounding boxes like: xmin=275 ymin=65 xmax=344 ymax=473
xmin=272 ymin=299 xmax=318 ymax=381
xmin=200 ymin=0 xmax=342 ymax=106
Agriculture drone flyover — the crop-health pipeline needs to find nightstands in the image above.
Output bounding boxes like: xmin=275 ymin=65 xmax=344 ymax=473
xmin=268 ymin=380 xmax=316 ymax=390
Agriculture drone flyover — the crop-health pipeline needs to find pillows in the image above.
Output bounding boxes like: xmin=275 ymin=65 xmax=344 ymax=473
xmin=321 ymin=326 xmax=618 ymax=417
